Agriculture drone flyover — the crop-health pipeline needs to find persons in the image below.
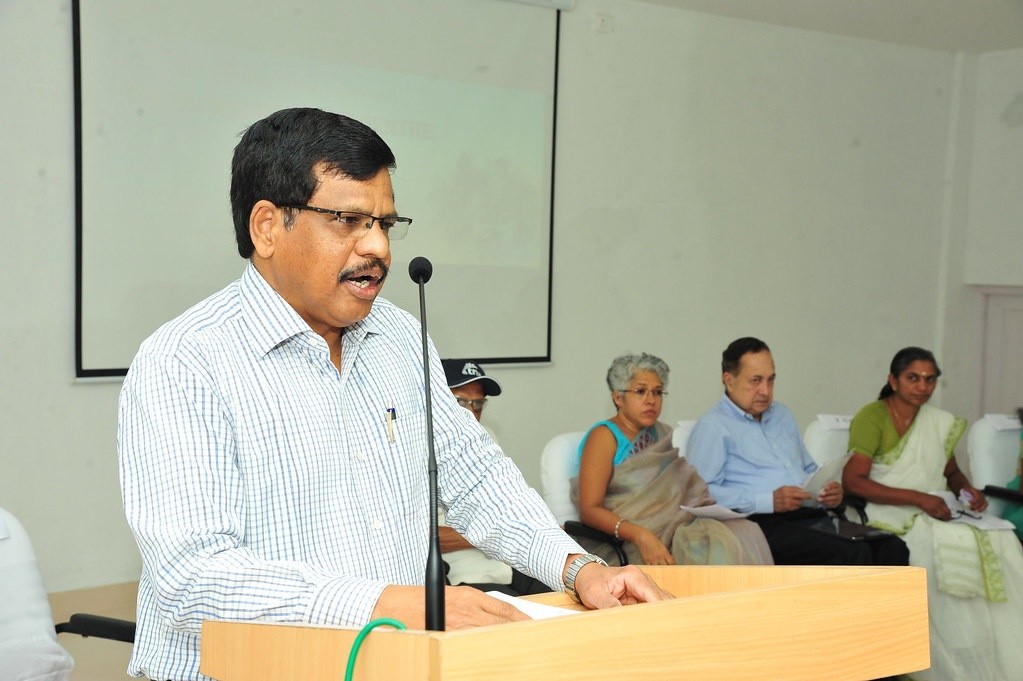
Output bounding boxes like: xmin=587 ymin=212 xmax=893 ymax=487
xmin=438 ymin=359 xmax=553 ymax=596
xmin=577 ymin=353 xmax=773 ymax=566
xmin=116 ymin=107 xmax=679 ymax=681
xmin=686 ymin=337 xmax=910 ymax=566
xmin=841 ymin=347 xmax=1023 ymax=681
xmin=1005 ymin=407 xmax=1023 ymax=542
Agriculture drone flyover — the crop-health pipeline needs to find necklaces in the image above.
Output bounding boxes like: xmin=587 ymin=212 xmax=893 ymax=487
xmin=890 ymin=397 xmax=911 ymax=426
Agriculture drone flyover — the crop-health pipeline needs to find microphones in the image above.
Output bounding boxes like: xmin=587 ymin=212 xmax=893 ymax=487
xmin=408 ymin=256 xmax=446 ymax=632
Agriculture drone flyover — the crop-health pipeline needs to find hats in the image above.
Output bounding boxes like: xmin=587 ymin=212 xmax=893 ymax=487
xmin=442 ymin=360 xmax=501 ymax=396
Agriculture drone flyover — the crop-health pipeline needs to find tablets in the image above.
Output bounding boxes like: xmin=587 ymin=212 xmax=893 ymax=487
xmin=787 ymin=515 xmax=894 ymax=541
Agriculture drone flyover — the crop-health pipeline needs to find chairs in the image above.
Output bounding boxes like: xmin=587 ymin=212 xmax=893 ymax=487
xmin=540 ymin=431 xmax=583 ymax=525
xmin=967 ymin=415 xmax=1021 ymax=517
xmin=804 ymin=414 xmax=851 ymax=488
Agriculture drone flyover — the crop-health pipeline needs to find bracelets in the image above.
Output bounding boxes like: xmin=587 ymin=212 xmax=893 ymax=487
xmin=615 ymin=519 xmax=626 ymax=541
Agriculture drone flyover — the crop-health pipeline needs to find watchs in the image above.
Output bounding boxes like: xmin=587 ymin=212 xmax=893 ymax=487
xmin=565 ymin=554 xmax=609 ymax=605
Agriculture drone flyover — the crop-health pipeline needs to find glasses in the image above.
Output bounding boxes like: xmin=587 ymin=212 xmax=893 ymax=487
xmin=619 ymin=388 xmax=668 ymax=398
xmin=276 ymin=203 xmax=413 ymax=240
xmin=456 ymin=398 xmax=488 ymax=412
